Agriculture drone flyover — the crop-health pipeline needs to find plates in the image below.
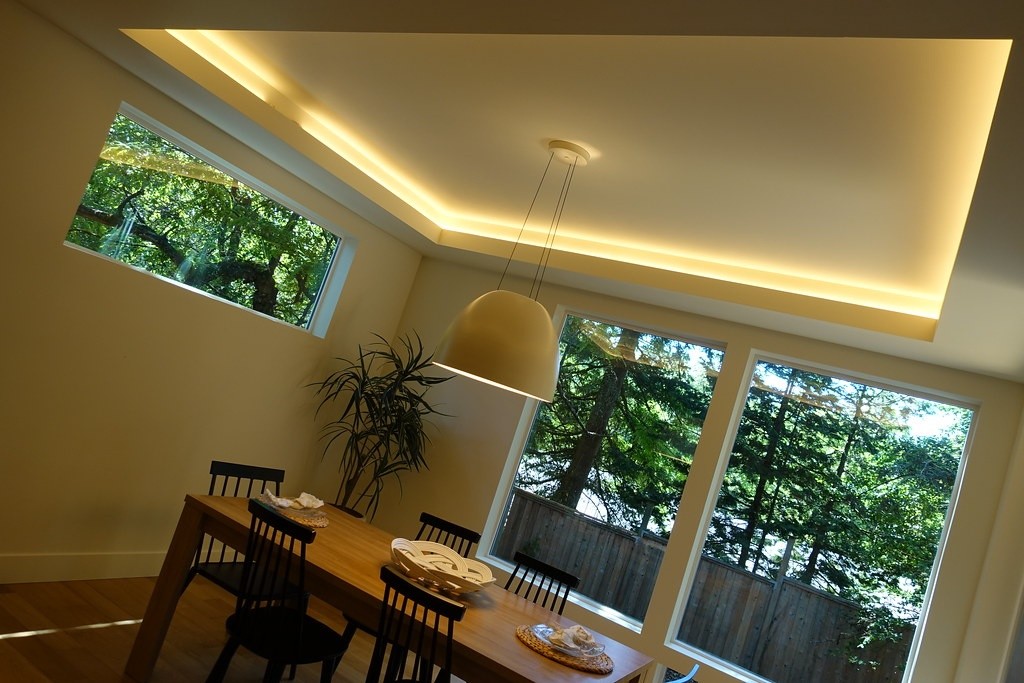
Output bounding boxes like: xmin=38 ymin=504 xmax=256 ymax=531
xmin=260 ymin=494 xmax=327 ymax=520
xmin=528 ymin=620 xmax=605 ymax=659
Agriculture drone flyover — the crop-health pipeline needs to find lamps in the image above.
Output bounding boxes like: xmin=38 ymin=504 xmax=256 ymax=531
xmin=429 ymin=139 xmax=591 ymax=402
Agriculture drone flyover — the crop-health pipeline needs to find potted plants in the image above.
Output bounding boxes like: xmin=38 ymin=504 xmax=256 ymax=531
xmin=300 ymin=326 xmax=459 ymax=525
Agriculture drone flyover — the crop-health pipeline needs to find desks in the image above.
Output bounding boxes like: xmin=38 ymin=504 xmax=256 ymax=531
xmin=121 ymin=494 xmax=655 ymax=683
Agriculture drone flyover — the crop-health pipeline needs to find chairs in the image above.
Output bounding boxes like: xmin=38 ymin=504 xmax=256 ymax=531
xmin=182 ymin=461 xmax=700 ymax=683
